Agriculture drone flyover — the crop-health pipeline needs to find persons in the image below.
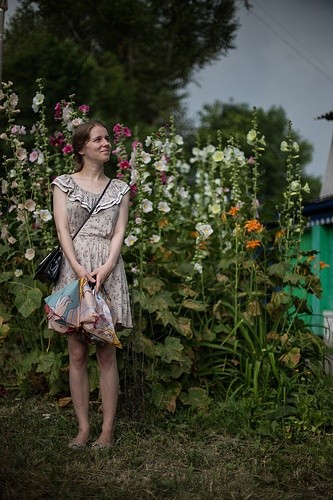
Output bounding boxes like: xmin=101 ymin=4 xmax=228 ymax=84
xmin=42 ymin=119 xmax=138 ymax=457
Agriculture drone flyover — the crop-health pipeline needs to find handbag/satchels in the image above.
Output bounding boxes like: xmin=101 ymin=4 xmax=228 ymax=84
xmin=33 ymin=244 xmax=62 ymax=286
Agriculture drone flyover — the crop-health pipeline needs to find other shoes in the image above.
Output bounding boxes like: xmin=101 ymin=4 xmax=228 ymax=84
xmin=91 ymin=433 xmax=115 ymax=451
xmin=68 ymin=434 xmax=92 ymax=449
